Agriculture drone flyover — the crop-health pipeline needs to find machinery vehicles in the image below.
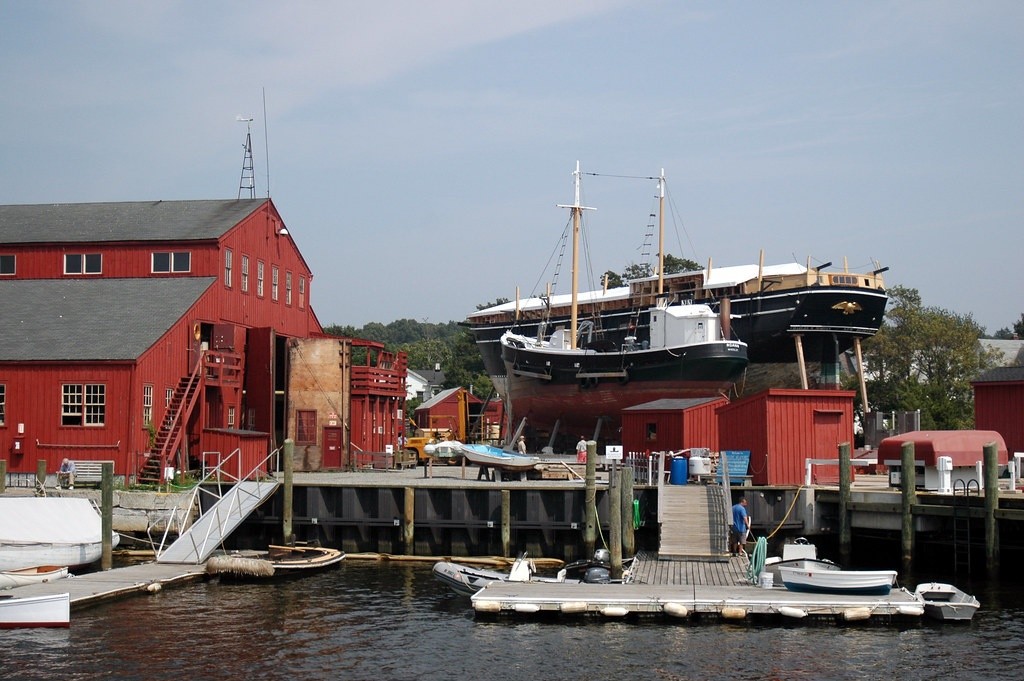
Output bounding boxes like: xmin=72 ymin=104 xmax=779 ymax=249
xmin=403 ymin=388 xmax=465 ymax=465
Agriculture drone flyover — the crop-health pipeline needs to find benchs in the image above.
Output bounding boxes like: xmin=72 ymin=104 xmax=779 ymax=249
xmin=57 ymin=460 xmax=115 ymax=489
xmin=396 ymin=460 xmax=418 ymax=471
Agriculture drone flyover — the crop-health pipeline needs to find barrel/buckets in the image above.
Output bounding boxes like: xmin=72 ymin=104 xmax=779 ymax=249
xmin=761 ymin=573 xmax=773 ymax=588
xmin=670 ymin=457 xmax=687 ymax=484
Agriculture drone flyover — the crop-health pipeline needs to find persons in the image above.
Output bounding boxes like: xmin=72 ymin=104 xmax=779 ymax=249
xmin=55 ymin=458 xmax=77 ymax=490
xmin=398 ymin=432 xmax=408 ymax=450
xmin=518 ymin=436 xmax=526 ymax=454
xmin=576 ymin=436 xmax=587 ymax=463
xmin=732 ymin=497 xmax=750 ymax=557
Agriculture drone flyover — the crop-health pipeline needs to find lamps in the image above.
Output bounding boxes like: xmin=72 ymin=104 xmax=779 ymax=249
xmin=279 ymin=229 xmax=288 ymax=238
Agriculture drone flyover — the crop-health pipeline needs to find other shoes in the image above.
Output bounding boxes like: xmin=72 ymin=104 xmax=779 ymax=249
xmin=733 ymin=552 xmax=736 ymax=557
xmin=739 ymin=553 xmax=746 ymax=557
xmin=69 ymin=486 xmax=73 ymax=490
xmin=55 ymin=486 xmax=62 ymax=490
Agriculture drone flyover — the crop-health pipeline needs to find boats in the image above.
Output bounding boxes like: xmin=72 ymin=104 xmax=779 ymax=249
xmin=462 ymin=445 xmax=539 ymax=473
xmin=0 ymin=498 xmax=120 ymax=566
xmin=914 ymin=582 xmax=981 ymax=620
xmin=205 ymin=533 xmax=346 ymax=578
xmin=433 ymin=549 xmax=629 ymax=598
xmin=0 ymin=594 xmax=69 ymax=627
xmin=765 ymin=537 xmax=842 ymax=587
xmin=457 ymin=160 xmax=889 ymax=450
xmin=0 ymin=566 xmax=69 ymax=590
xmin=778 ymin=567 xmax=899 ymax=596
